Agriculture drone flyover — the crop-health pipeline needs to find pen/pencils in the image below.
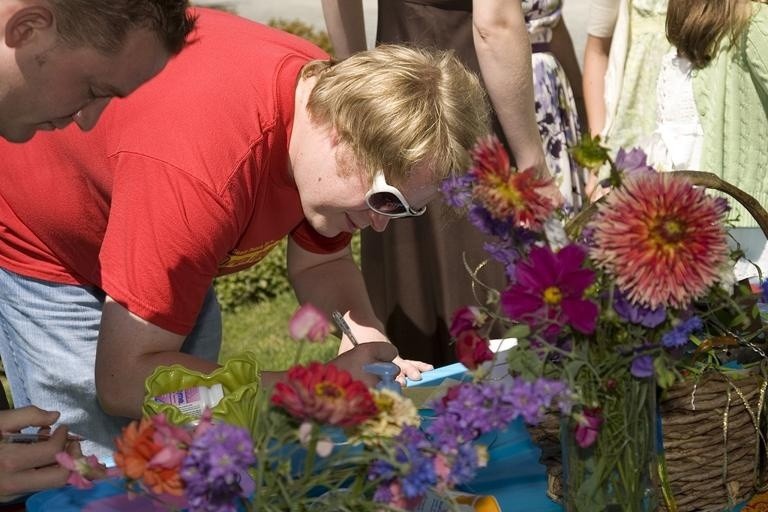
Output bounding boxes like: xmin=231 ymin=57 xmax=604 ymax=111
xmin=331 ymin=310 xmax=358 ymax=347
xmin=1 ymin=432 xmax=86 ymax=443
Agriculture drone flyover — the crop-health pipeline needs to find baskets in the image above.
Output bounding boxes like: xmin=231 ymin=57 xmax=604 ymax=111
xmin=527 ymin=170 xmax=767 ymax=511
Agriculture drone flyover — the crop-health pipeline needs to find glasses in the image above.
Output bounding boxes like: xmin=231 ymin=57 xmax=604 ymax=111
xmin=365 ymin=160 xmax=426 ymax=219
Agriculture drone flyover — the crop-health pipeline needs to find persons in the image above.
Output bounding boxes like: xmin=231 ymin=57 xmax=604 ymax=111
xmin=0 ymin=10 xmax=492 ymax=468
xmin=322 ymin=0 xmax=586 ymax=369
xmin=0 ymin=0 xmax=203 ymax=503
xmin=582 ymin=0 xmax=768 ymax=228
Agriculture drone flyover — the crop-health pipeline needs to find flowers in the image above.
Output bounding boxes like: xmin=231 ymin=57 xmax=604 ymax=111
xmin=57 ymin=146 xmax=767 ymax=510
